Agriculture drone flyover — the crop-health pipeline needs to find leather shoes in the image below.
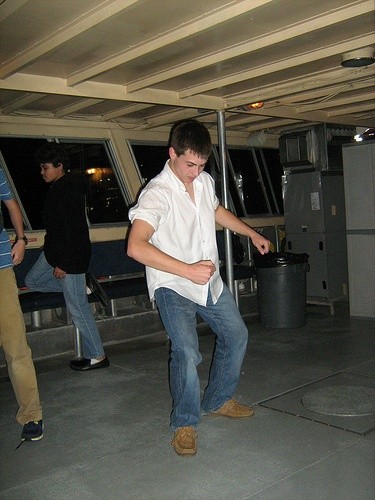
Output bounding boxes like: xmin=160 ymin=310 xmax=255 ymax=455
xmin=69 ymin=358 xmax=109 ymax=371
xmin=84 ymin=272 xmax=110 ymax=309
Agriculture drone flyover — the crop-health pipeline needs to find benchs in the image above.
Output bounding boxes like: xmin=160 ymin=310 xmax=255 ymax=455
xmin=12 ymin=230 xmax=254 ymax=359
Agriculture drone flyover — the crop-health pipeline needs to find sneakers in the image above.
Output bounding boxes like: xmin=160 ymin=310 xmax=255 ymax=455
xmin=21 ymin=418 xmax=45 ymax=441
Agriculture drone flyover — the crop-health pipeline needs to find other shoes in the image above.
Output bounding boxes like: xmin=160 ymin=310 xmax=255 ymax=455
xmin=207 ymin=400 xmax=254 ymax=418
xmin=172 ymin=425 xmax=198 ymax=457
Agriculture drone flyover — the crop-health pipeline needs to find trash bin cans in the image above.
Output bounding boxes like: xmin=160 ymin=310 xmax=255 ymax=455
xmin=253 ymin=251 xmax=310 ymax=329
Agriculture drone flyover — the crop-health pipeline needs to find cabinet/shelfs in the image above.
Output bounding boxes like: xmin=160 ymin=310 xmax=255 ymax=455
xmin=281 ymin=140 xmax=375 ymax=319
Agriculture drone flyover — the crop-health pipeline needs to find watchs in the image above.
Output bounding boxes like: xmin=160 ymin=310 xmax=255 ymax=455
xmin=15 ymin=235 xmax=29 ymax=247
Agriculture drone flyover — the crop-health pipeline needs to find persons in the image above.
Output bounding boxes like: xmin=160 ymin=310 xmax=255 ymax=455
xmin=24 ymin=141 xmax=110 ymax=371
xmin=125 ymin=117 xmax=272 ymax=456
xmin=0 ymin=164 xmax=46 ymax=443
